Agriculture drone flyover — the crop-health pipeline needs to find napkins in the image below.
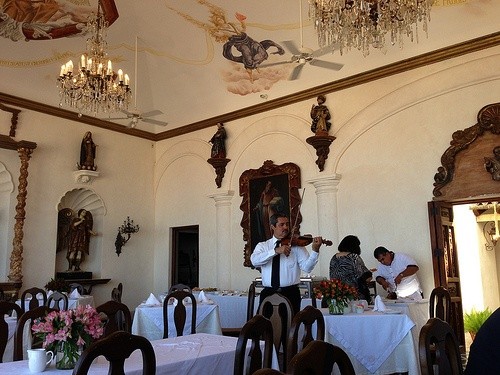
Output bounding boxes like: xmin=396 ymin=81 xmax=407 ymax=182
xmin=197 ymin=290 xmax=208 ymax=302
xmin=145 ymin=293 xmax=160 ymax=306
xmin=70 ymin=288 xmax=82 ymax=299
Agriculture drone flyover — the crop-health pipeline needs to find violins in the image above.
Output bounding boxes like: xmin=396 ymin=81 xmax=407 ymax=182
xmin=280 ymin=230 xmax=332 ymax=247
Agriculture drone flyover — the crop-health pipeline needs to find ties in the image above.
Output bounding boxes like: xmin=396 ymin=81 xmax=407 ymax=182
xmin=271 ymin=240 xmax=281 ymax=292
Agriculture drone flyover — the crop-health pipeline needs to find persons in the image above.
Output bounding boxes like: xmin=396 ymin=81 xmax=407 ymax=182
xmin=330 ymin=235 xmax=374 ymax=305
xmin=66 ymin=209 xmax=97 ymax=271
xmin=80 ymin=131 xmax=96 ymax=166
xmin=311 ymin=95 xmax=331 ymax=132
xmin=250 ymin=213 xmax=322 ymax=373
xmin=374 ymin=246 xmax=424 ymax=299
xmin=211 ymin=121 xmax=226 ymax=158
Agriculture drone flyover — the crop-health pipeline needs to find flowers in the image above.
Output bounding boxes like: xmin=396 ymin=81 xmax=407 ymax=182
xmin=312 ymin=278 xmax=363 ymax=306
xmin=32 ymin=306 xmax=109 ymax=362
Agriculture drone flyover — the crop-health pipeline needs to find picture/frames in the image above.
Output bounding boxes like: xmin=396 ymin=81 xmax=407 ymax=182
xmin=239 ymin=160 xmax=301 ymax=272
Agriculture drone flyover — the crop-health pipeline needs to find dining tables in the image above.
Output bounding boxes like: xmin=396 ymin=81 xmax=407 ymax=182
xmin=0 ymin=334 xmax=280 ymax=375
xmin=308 ymin=304 xmax=420 ymax=375
xmin=192 ymin=290 xmax=262 ymax=335
xmin=11 ymin=295 xmax=94 ymax=313
xmin=132 ymin=300 xmax=223 ymax=341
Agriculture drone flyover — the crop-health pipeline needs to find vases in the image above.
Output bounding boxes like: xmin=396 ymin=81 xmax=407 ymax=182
xmin=52 ymin=338 xmax=80 ymax=370
xmin=327 ymin=297 xmax=343 ymax=315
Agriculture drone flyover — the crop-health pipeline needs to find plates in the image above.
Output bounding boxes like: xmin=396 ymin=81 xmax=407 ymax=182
xmin=202 ymin=301 xmax=214 ymax=305
xmin=383 ymin=311 xmax=402 ymax=314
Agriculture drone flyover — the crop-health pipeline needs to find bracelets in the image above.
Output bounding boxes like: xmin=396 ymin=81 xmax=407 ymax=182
xmin=400 ymin=273 xmax=403 ymax=276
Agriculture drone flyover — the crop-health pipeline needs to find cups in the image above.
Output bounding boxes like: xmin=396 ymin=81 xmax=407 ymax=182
xmin=26 ymin=349 xmax=54 ymax=373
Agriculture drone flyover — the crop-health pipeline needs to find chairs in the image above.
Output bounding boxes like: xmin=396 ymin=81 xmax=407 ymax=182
xmin=0 ymin=282 xmax=463 ymax=375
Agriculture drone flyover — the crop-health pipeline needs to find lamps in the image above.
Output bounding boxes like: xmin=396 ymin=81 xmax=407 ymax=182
xmin=308 ymin=0 xmax=434 ymax=55
xmin=115 ymin=216 xmax=140 ymax=257
xmin=55 ymin=0 xmax=132 ymax=116
